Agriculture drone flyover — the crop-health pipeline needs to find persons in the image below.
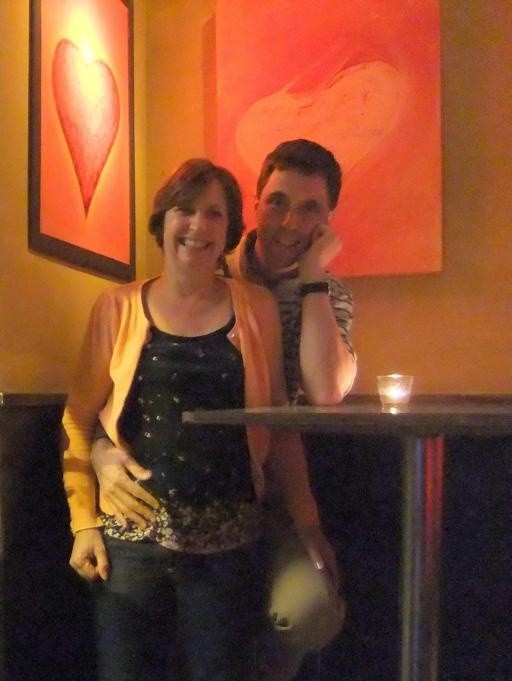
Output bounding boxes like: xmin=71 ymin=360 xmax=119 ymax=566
xmin=58 ymin=157 xmax=348 ymax=680
xmin=88 ymin=138 xmax=364 ymax=679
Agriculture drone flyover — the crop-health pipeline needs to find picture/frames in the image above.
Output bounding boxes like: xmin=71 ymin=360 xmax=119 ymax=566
xmin=28 ymin=0 xmax=135 ymax=284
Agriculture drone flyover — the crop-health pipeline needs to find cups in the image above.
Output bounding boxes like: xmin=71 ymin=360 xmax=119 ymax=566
xmin=375 ymin=374 xmax=415 ymax=408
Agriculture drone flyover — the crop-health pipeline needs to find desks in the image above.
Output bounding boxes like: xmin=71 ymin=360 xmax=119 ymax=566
xmin=182 ymin=403 xmax=512 ymax=681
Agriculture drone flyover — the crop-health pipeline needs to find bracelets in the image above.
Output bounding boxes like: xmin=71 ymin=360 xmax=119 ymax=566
xmin=297 ymin=280 xmax=332 ymax=297
xmin=91 ymin=433 xmax=109 ymax=445
